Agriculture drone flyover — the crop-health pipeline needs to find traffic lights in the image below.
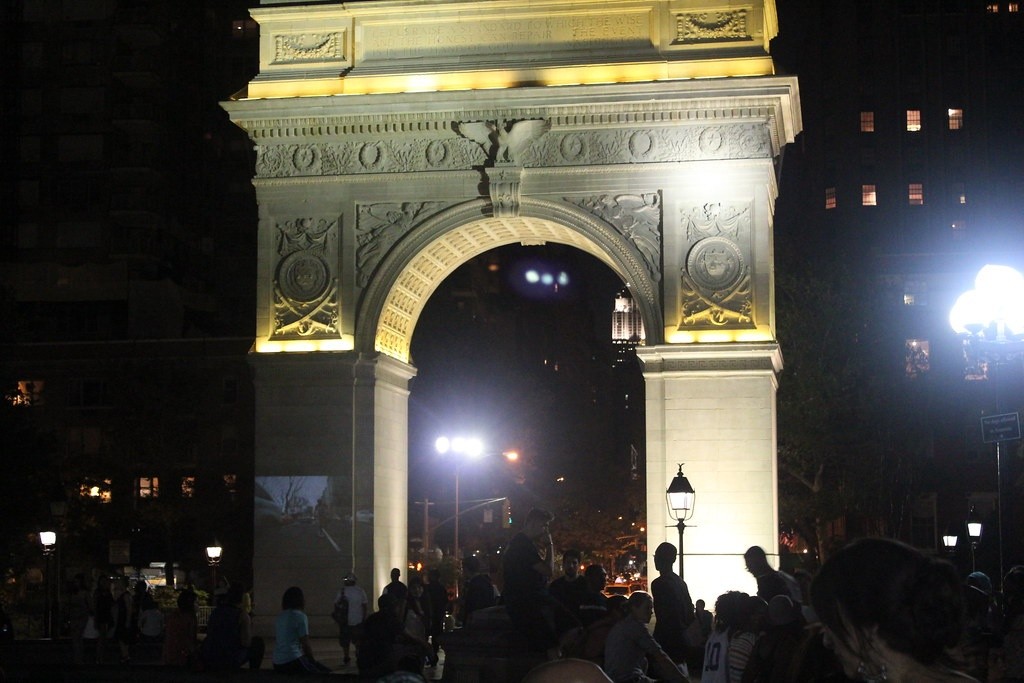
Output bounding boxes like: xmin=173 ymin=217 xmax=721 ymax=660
xmin=501 ymin=500 xmax=513 ymax=529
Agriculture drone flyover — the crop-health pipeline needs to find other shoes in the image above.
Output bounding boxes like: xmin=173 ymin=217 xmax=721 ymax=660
xmin=340 ymin=657 xmax=351 ymax=666
xmin=120 ymin=656 xmax=130 ymax=664
xmin=94 ymin=656 xmax=105 ymax=667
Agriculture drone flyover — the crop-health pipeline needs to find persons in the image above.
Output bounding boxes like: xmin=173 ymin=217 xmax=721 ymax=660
xmin=745 ymin=546 xmax=802 ymax=606
xmin=271 ymin=586 xmax=331 ymax=674
xmin=333 ymin=573 xmax=368 ymax=665
xmin=461 ymin=556 xmax=494 ymax=626
xmin=140 ymin=602 xmax=164 ymax=643
xmin=423 ymin=569 xmax=447 ymax=668
xmin=997 ymin=566 xmax=1024 ymax=683
xmin=403 ymin=578 xmax=432 ymax=642
xmin=160 ymin=590 xmax=199 ymax=666
xmin=356 ymin=594 xmax=439 ymax=683
xmin=808 ymin=537 xmax=978 ymax=683
xmin=501 ymin=507 xmax=554 ymax=671
xmin=202 ymin=576 xmax=265 ymax=672
xmin=70 ymin=572 xmax=146 ymax=665
xmin=698 ymin=592 xmax=866 ymax=683
xmin=603 ymin=592 xmax=688 ymax=683
xmin=695 ymin=600 xmax=713 ymax=636
xmin=385 ymin=568 xmax=407 ymax=599
xmin=650 ymin=543 xmax=695 ymax=666
xmin=546 ymin=549 xmax=628 ymax=671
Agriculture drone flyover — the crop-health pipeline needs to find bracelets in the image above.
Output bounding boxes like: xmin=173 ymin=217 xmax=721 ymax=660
xmin=545 ymin=542 xmax=553 ymax=547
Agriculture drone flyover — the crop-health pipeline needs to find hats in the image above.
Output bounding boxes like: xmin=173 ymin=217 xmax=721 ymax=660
xmin=342 ymin=573 xmax=356 ymax=582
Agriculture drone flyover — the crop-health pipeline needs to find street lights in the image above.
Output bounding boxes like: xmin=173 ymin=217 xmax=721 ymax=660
xmin=453 ymin=448 xmax=521 ymax=601
xmin=963 ymin=505 xmax=983 ymax=573
xmin=203 ymin=535 xmax=224 ymax=605
xmin=37 ymin=520 xmax=58 ymax=637
xmin=663 ymin=461 xmax=698 ymax=581
xmin=942 ymin=521 xmax=959 ymax=564
xmin=949 ymin=264 xmax=1024 ymax=618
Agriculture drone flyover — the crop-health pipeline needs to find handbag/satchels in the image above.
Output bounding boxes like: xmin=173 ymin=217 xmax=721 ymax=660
xmin=331 ymin=587 xmax=348 ymax=625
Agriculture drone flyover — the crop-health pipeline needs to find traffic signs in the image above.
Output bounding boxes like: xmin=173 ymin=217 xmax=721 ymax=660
xmin=980 ymin=412 xmax=1022 ymax=444
